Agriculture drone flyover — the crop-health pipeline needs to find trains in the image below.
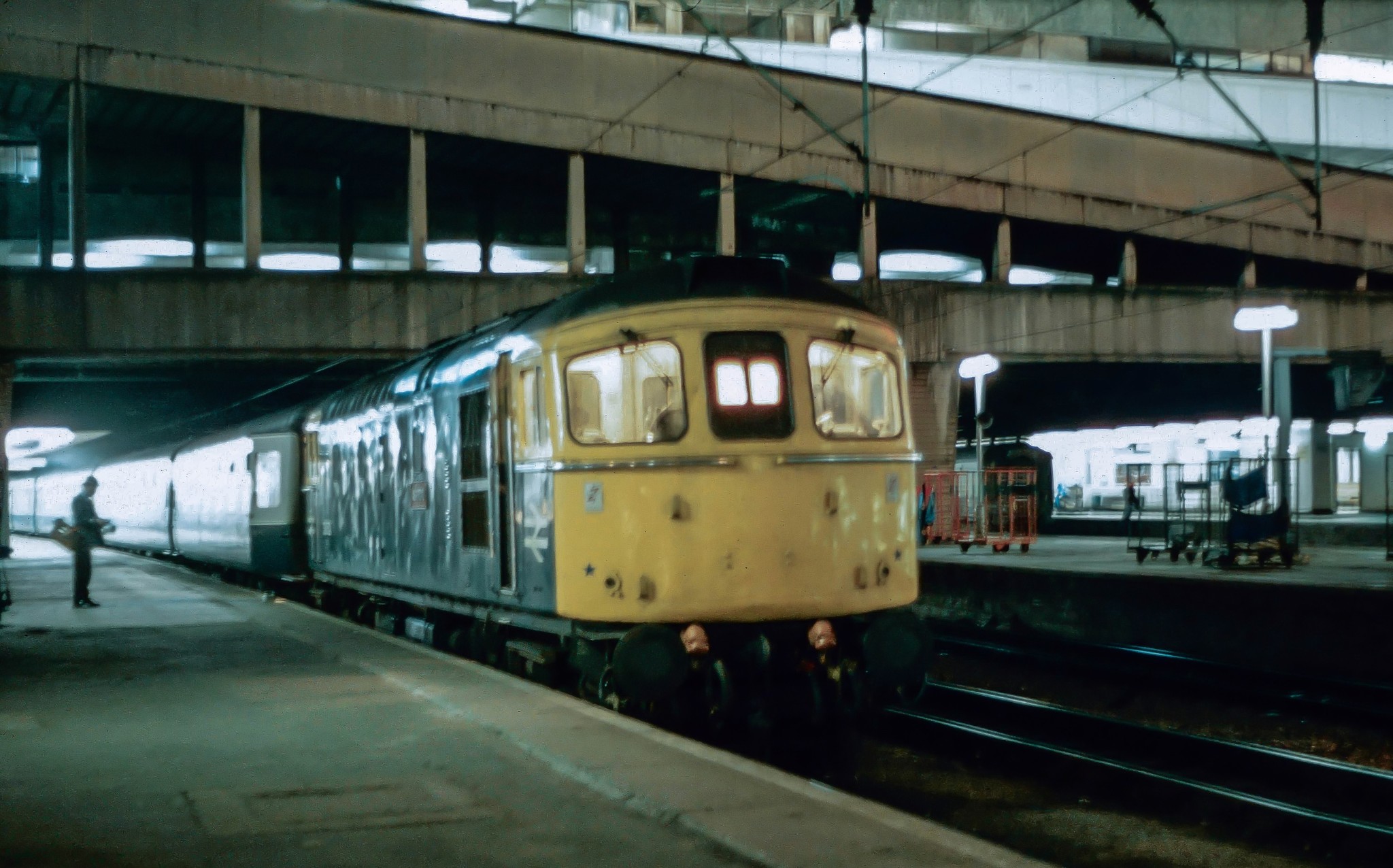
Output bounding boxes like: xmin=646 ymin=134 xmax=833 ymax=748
xmin=0 ymin=250 xmax=920 ymax=726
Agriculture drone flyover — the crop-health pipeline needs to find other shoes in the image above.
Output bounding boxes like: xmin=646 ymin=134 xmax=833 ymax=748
xmin=83 ymin=596 xmax=99 ymax=607
xmin=72 ymin=600 xmax=80 ymax=608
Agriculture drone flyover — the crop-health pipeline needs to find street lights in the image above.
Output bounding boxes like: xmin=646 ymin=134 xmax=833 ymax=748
xmin=1234 ymin=303 xmax=1297 ymax=514
xmin=959 ymin=352 xmax=1000 ymax=535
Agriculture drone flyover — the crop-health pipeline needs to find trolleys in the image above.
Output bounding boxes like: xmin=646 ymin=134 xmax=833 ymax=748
xmin=1125 ymin=462 xmax=1209 ymax=563
xmin=1204 ymin=458 xmax=1301 ymax=572
xmin=926 ymin=464 xmax=1037 ymax=555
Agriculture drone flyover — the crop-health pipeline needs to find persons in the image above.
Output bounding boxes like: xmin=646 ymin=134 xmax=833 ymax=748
xmin=1121 ymin=482 xmax=1136 ymax=524
xmin=71 ymin=475 xmax=111 ymax=608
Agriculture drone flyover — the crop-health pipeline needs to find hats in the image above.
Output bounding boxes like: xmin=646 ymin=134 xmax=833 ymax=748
xmin=82 ymin=476 xmax=100 ymax=487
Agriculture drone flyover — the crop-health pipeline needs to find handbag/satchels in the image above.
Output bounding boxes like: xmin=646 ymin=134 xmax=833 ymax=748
xmin=48 ymin=518 xmax=83 ymax=550
